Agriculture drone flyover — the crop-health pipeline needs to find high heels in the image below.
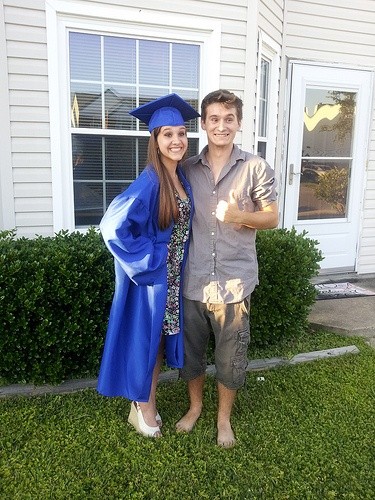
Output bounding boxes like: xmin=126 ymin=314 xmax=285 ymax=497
xmin=127 ymin=402 xmax=164 ymax=442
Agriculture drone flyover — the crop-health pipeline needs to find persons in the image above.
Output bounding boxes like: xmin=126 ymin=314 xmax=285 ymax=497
xmin=98 ymin=92 xmax=194 ymax=440
xmin=176 ymin=89 xmax=281 ymax=449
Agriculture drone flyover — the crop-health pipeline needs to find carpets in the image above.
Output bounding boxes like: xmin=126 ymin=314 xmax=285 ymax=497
xmin=313 ymin=282 xmax=375 ymax=300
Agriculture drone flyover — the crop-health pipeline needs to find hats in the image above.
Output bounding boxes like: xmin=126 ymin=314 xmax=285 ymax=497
xmin=129 ymin=93 xmax=202 ymax=130
